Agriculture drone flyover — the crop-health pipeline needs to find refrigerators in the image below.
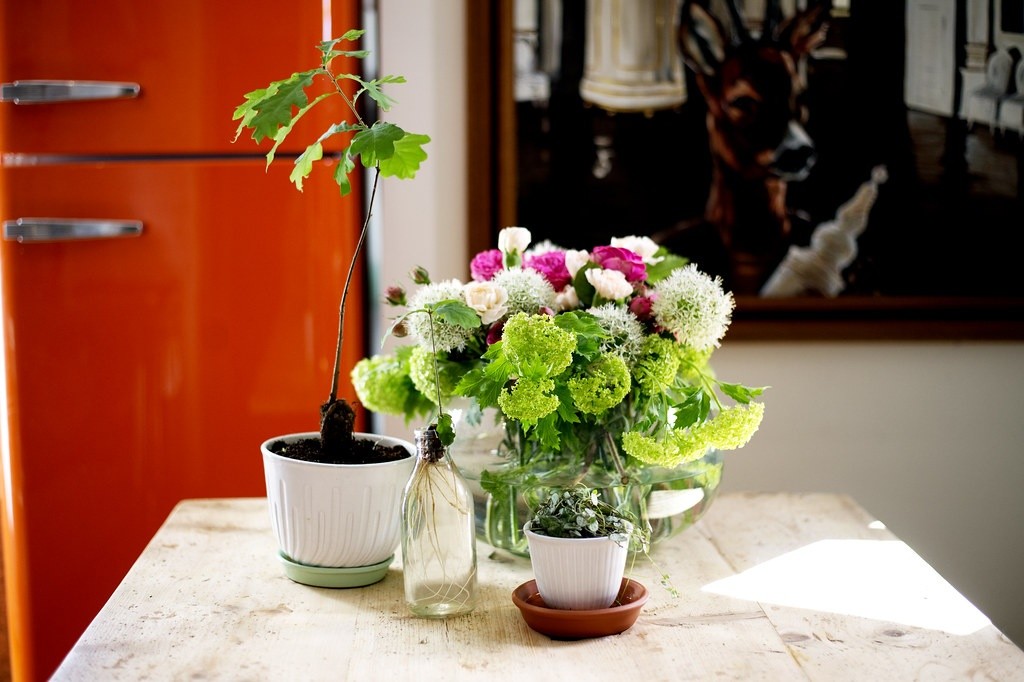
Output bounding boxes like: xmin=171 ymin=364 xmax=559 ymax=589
xmin=0 ymin=0 xmax=371 ymax=682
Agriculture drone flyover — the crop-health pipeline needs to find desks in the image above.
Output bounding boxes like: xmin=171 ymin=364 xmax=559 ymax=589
xmin=51 ymin=499 xmax=1024 ymax=682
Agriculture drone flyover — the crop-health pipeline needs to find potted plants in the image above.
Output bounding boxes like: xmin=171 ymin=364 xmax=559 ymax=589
xmin=230 ymin=26 xmax=418 ymax=565
xmin=522 ymin=514 xmax=633 ymax=610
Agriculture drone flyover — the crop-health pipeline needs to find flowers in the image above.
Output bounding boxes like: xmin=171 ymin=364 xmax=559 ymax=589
xmin=352 ymin=227 xmax=771 ymax=532
